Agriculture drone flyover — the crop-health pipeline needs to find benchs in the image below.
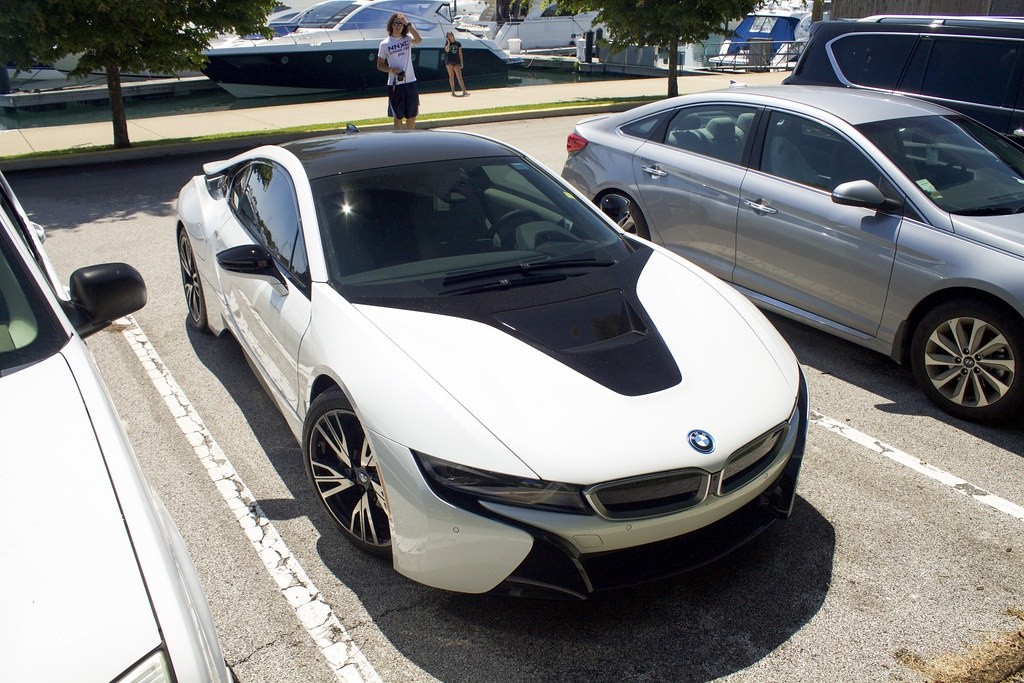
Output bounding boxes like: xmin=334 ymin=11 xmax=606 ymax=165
xmin=670 ymin=111 xmax=755 ymax=164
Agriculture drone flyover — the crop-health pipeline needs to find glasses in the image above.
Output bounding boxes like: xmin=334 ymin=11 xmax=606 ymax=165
xmin=394 ymin=22 xmax=402 ymax=27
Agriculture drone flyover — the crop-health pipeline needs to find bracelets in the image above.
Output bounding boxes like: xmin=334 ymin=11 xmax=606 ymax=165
xmin=406 ymin=22 xmax=411 ymax=27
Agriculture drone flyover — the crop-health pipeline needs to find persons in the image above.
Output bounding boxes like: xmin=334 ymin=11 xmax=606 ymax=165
xmin=444 ymin=31 xmax=471 ymax=97
xmin=376 ymin=13 xmax=422 ymax=130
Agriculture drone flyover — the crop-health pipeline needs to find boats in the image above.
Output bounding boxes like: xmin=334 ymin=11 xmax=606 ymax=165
xmin=703 ymin=7 xmax=811 ymax=74
xmin=455 ymin=0 xmax=633 ymax=51
xmin=200 ymin=0 xmax=524 ymax=101
xmin=8 ymin=26 xmax=233 ymax=91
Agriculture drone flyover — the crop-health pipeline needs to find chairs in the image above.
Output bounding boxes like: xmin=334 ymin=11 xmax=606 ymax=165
xmin=767 ymin=137 xmax=820 ymax=186
xmin=833 ymin=126 xmax=899 ymax=182
xmin=411 ymin=180 xmax=488 ymax=256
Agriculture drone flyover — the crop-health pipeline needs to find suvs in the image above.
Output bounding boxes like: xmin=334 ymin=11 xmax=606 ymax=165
xmin=782 ymin=15 xmax=1024 ymax=168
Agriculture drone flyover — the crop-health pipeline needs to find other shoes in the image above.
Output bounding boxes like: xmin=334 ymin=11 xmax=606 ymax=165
xmin=452 ymin=92 xmax=456 ymax=96
xmin=463 ymin=92 xmax=470 ymax=96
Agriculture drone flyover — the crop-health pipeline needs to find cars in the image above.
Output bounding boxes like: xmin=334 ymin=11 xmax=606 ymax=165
xmin=0 ymin=170 xmax=239 ymax=683
xmin=176 ymin=121 xmax=808 ymax=604
xmin=562 ymin=81 xmax=1024 ymax=419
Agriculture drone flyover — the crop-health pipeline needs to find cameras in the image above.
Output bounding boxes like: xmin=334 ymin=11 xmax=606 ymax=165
xmin=397 ymin=71 xmax=405 ymax=82
